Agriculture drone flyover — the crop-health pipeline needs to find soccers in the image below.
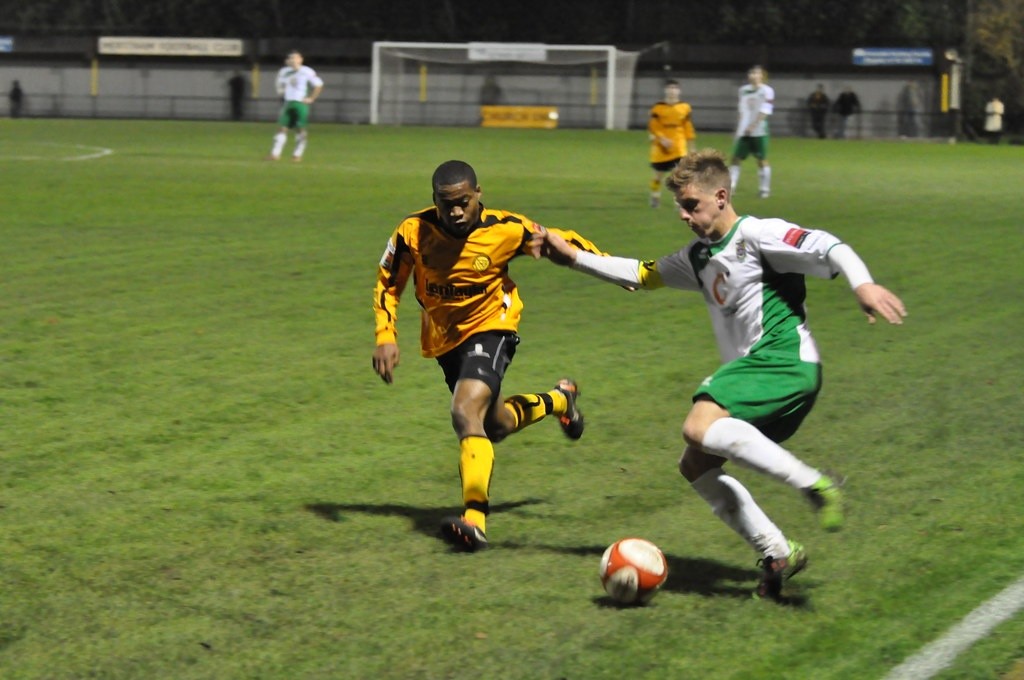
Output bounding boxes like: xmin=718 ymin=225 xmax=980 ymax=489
xmin=598 ymin=537 xmax=668 ymax=607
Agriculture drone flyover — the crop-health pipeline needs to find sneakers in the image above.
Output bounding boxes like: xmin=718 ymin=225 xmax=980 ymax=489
xmin=554 ymin=377 xmax=584 ymax=441
xmin=751 ymin=540 xmax=808 ymax=600
xmin=440 ymin=515 xmax=490 ymax=550
xmin=811 ymin=472 xmax=850 ymax=533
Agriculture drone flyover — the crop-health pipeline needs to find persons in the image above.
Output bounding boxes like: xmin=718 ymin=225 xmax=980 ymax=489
xmin=554 ymin=146 xmax=908 ymax=599
xmin=372 ymin=156 xmax=663 ymax=552
xmin=981 ymin=95 xmax=1005 ymax=144
xmin=725 ymin=64 xmax=777 ymax=201
xmin=952 ymin=83 xmax=982 ymax=146
xmin=897 ymin=82 xmax=919 ymax=139
xmin=224 ymin=68 xmax=245 ymax=122
xmin=268 ymin=48 xmax=324 ymax=159
xmin=807 ymin=82 xmax=833 ymax=140
xmin=648 ymin=79 xmax=697 ymax=210
xmin=477 ymin=75 xmax=504 ymax=124
xmin=8 ymin=79 xmax=25 ymax=117
xmin=831 ymin=85 xmax=863 ymax=143
